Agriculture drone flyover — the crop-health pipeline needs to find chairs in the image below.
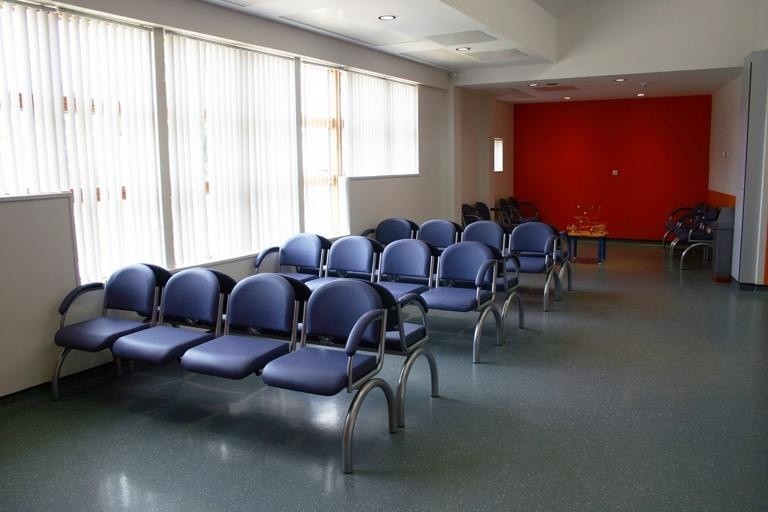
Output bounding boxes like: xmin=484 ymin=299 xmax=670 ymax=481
xmin=256 ymin=201 xmax=570 ymax=365
xmin=53 ymin=262 xmax=438 ymax=474
xmin=671 ymin=206 xmax=722 ymax=270
xmin=660 ymin=201 xmax=709 ymax=258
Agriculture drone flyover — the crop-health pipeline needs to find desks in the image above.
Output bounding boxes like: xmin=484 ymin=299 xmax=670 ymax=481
xmin=564 ymin=228 xmax=610 ymax=266
